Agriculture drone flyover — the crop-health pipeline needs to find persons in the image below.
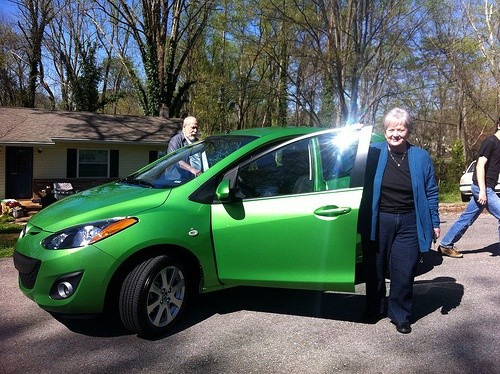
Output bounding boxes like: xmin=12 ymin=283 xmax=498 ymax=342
xmin=437 ymin=117 xmax=500 ymax=259
xmin=342 ymin=108 xmax=439 ymax=334
xmin=165 ymin=116 xmax=202 ymax=182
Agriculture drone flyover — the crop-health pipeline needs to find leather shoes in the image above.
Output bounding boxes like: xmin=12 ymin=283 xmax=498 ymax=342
xmin=397 ymin=323 xmax=412 ymax=334
xmin=362 ymin=311 xmax=386 ymax=324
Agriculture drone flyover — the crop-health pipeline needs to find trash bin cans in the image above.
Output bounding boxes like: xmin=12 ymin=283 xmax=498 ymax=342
xmin=54 ymin=182 xmax=74 ymax=201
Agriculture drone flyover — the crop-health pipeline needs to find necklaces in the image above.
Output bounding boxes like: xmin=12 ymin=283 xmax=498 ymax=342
xmin=388 ymin=145 xmax=407 ymax=166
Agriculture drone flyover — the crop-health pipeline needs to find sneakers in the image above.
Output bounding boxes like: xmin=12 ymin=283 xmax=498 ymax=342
xmin=439 ymin=244 xmax=463 ymax=258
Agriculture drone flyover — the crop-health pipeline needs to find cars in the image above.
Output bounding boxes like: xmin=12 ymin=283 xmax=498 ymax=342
xmin=13 ymin=122 xmax=388 ymax=336
xmin=458 ymin=161 xmax=500 ymax=202
